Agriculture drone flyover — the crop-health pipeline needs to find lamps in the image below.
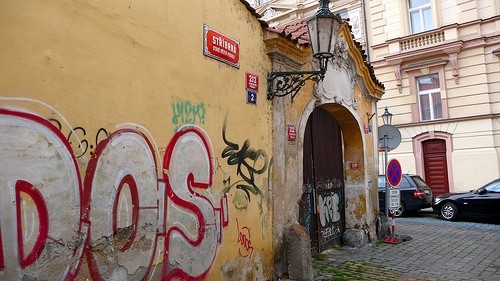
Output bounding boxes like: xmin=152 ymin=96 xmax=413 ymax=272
xmin=267 ymin=0 xmax=342 ymax=101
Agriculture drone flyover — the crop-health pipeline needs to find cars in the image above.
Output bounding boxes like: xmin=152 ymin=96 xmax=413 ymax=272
xmin=431 ymin=177 xmax=500 ymax=225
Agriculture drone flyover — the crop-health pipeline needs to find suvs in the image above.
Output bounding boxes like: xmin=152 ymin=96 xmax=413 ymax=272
xmin=378 ymin=172 xmax=433 ymax=218
